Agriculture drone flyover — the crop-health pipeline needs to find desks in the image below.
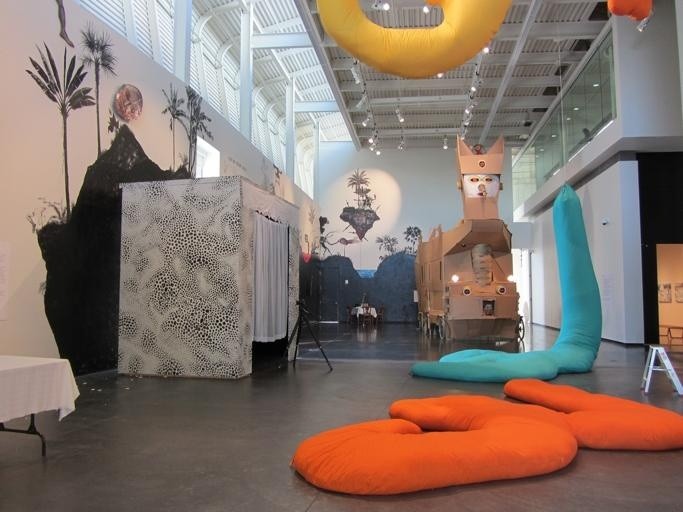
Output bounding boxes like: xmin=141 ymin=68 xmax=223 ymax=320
xmin=0 ymin=353 xmax=71 ymax=460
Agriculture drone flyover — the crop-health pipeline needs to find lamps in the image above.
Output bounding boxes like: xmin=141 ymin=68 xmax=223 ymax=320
xmin=350 ymin=62 xmax=382 ymax=157
xmin=460 ymin=45 xmax=491 ymax=148
xmin=395 ymin=106 xmax=407 ymax=154
xmin=441 ymin=136 xmax=450 ymax=151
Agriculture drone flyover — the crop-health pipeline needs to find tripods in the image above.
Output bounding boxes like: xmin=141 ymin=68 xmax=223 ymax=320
xmin=278 ymin=299 xmax=333 ymax=371
